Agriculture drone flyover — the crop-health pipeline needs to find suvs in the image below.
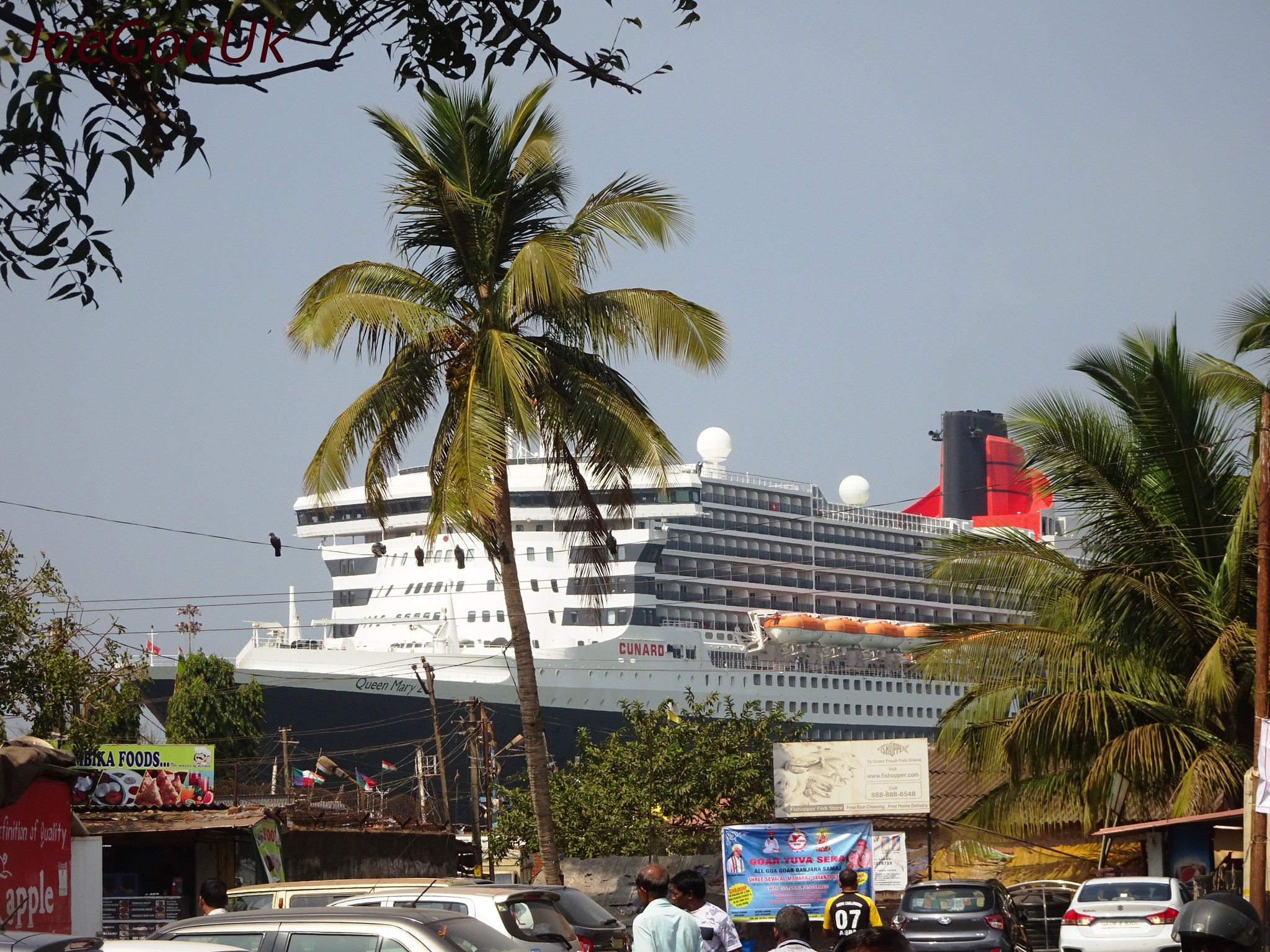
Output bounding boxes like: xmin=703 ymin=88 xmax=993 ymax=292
xmin=990 ymin=880 xmax=1084 ymax=950
xmin=892 ymin=878 xmax=1034 ymax=951
xmin=327 ymin=891 xmax=587 ymax=952
xmin=446 ymin=883 xmax=630 ymax=952
xmin=153 ymin=908 xmax=537 ymax=952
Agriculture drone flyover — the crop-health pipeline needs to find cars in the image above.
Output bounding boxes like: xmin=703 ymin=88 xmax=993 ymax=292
xmin=1056 ymin=879 xmax=1195 ymax=951
xmin=444 ymin=869 xmax=521 ymax=886
xmin=0 ymin=932 xmax=248 ymax=952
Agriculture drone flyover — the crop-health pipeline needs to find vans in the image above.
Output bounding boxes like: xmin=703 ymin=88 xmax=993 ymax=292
xmin=225 ymin=874 xmax=474 ymax=911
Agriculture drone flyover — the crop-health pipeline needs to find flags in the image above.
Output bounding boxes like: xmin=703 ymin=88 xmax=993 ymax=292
xmin=355 ymin=769 xmax=377 ymax=793
xmin=382 ymin=760 xmax=399 ymax=775
xmin=310 ymin=771 xmax=326 ymax=785
xmin=292 ymin=767 xmax=313 ymax=787
xmin=146 ymin=639 xmax=161 ymax=656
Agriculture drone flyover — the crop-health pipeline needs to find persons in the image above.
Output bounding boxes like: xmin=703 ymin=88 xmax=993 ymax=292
xmin=822 ymin=868 xmax=916 ymax=952
xmin=765 ymin=905 xmax=820 ymax=952
xmin=198 ymin=878 xmax=229 ymax=916
xmin=632 ymin=865 xmax=743 ymax=952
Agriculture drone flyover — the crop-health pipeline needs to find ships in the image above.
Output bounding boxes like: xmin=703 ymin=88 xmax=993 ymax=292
xmin=110 ymin=411 xmax=1111 ymax=806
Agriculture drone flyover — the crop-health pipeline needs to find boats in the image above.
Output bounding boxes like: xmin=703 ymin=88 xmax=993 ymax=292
xmin=763 ymin=614 xmax=997 ymax=655
